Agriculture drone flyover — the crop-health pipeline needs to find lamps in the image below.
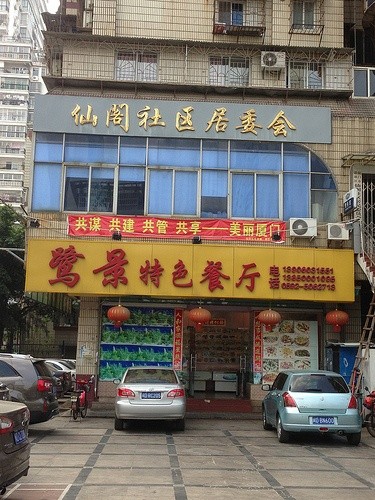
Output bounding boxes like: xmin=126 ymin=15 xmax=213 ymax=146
xmin=111 ymin=230 xmax=122 ymax=241
xmin=29 ymin=219 xmax=40 ymax=228
xmin=192 ymin=235 xmax=203 ymax=245
xmin=271 ymin=231 xmax=281 ymax=242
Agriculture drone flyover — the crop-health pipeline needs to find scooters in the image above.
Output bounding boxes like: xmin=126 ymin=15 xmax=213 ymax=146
xmin=68 ymin=373 xmax=96 ymax=423
xmin=362 ymin=386 xmax=375 ymax=439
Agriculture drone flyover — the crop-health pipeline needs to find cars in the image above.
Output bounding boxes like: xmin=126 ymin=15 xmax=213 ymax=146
xmin=261 ymin=368 xmax=363 ymax=446
xmin=0 ymin=400 xmax=31 ymax=496
xmin=112 ymin=366 xmax=186 ymax=432
xmin=0 ymin=351 xmax=77 ymax=424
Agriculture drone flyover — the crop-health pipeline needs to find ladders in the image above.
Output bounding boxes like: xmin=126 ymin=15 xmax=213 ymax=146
xmin=348 ymin=293 xmax=375 ymax=396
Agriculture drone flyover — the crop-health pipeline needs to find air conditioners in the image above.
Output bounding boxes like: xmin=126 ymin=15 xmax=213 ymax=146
xmin=32 ymin=75 xmax=38 ymax=81
xmin=328 ymin=222 xmax=349 ymax=241
xmin=261 ymin=50 xmax=284 ymax=68
xmin=290 ymin=217 xmax=317 ymax=238
